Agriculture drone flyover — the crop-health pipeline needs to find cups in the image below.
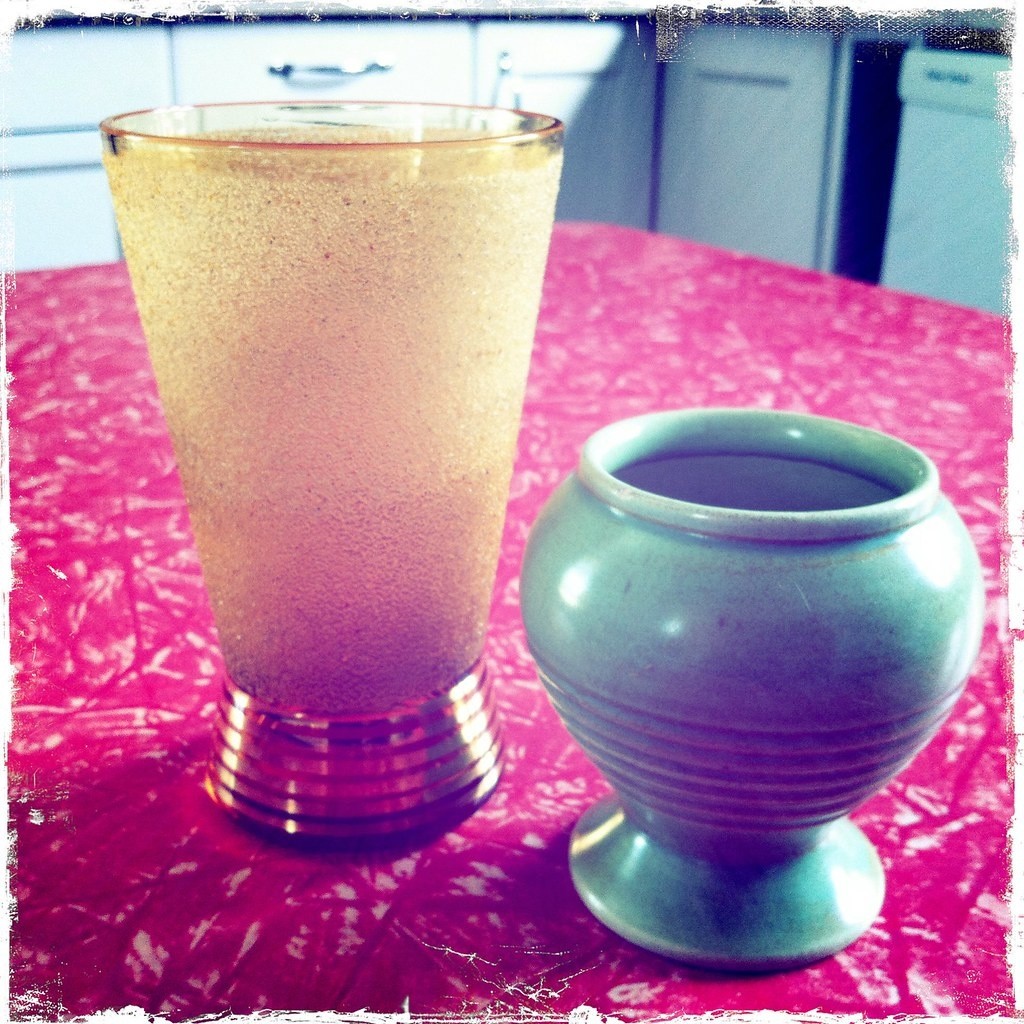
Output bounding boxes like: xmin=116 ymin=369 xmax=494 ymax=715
xmin=100 ymin=101 xmax=564 ymax=845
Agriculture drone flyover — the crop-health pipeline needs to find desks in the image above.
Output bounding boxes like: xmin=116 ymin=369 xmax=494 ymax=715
xmin=0 ymin=220 xmax=1024 ymax=1024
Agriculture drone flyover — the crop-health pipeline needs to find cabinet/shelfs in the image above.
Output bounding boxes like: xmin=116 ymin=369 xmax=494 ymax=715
xmin=479 ymin=20 xmax=653 ymax=235
xmin=649 ymin=28 xmax=839 ymax=269
xmin=0 ymin=18 xmax=477 ymax=266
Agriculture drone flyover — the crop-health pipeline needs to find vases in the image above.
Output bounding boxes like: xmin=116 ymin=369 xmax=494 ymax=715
xmin=518 ymin=407 xmax=984 ymax=967
